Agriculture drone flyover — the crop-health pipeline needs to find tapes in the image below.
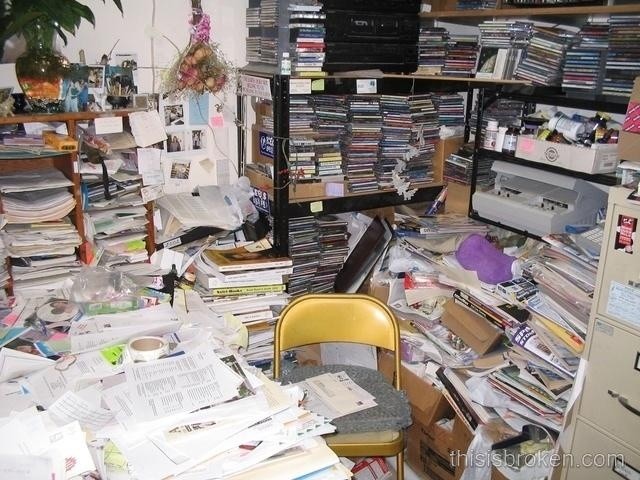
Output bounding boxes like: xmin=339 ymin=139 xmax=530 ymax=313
xmin=126 ymin=336 xmax=170 ymax=363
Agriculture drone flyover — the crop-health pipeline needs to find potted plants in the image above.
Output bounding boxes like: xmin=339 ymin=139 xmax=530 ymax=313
xmin=0 ymin=0 xmax=124 ymax=99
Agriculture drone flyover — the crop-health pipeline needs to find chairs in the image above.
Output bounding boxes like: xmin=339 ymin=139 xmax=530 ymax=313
xmin=273 ymin=293 xmax=405 ymax=480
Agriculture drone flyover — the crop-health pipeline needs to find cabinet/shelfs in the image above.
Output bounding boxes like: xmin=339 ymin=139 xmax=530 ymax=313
xmin=237 ymin=0 xmax=640 ymax=258
xmin=0 ymin=107 xmax=152 ymax=295
xmin=560 ymin=185 xmax=640 ymax=480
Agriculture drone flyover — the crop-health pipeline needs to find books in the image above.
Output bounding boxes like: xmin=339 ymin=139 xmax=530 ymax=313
xmin=188 ymin=243 xmax=260 ymax=286
xmin=152 ymin=185 xmax=259 ymax=244
xmin=261 ymin=91 xmax=524 ymax=195
xmin=0 ymin=289 xmax=379 ymax=480
xmin=0 ymin=172 xmax=80 ymax=290
xmin=262 ymin=213 xmax=352 ymax=289
xmin=412 ymin=19 xmax=640 ymax=97
xmin=245 ymin=0 xmax=325 ymax=74
xmin=81 ymin=167 xmax=151 ymax=265
xmin=457 ymin=0 xmax=563 ymax=10
xmin=352 ymin=213 xmax=598 ymax=480
